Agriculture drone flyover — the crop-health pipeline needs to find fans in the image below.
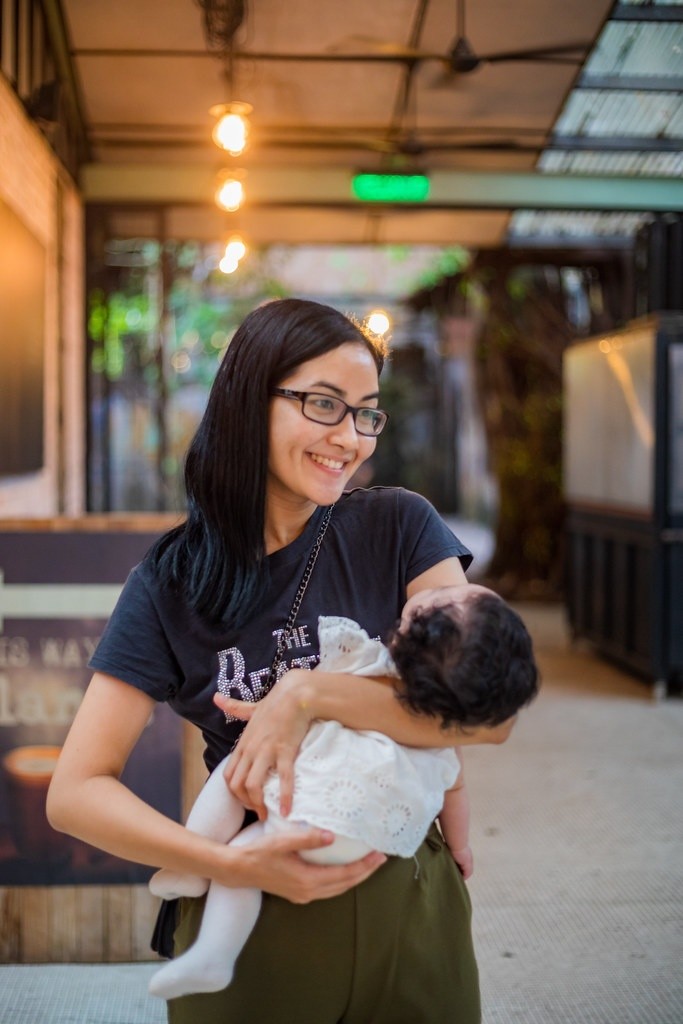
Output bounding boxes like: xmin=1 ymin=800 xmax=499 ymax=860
xmin=326 ymin=0 xmax=599 ymax=91
xmin=296 ymin=67 xmax=523 ymax=164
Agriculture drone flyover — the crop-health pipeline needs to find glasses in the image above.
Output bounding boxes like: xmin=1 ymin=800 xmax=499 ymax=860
xmin=273 ymin=387 xmax=388 ymax=438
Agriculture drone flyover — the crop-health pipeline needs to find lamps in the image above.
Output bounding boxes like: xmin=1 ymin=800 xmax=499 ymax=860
xmin=208 ymin=35 xmax=256 ymax=157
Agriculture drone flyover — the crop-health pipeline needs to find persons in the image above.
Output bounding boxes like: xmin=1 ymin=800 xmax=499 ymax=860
xmin=46 ymin=295 xmax=481 ymax=1024
xmin=148 ymin=580 xmax=546 ymax=1001
